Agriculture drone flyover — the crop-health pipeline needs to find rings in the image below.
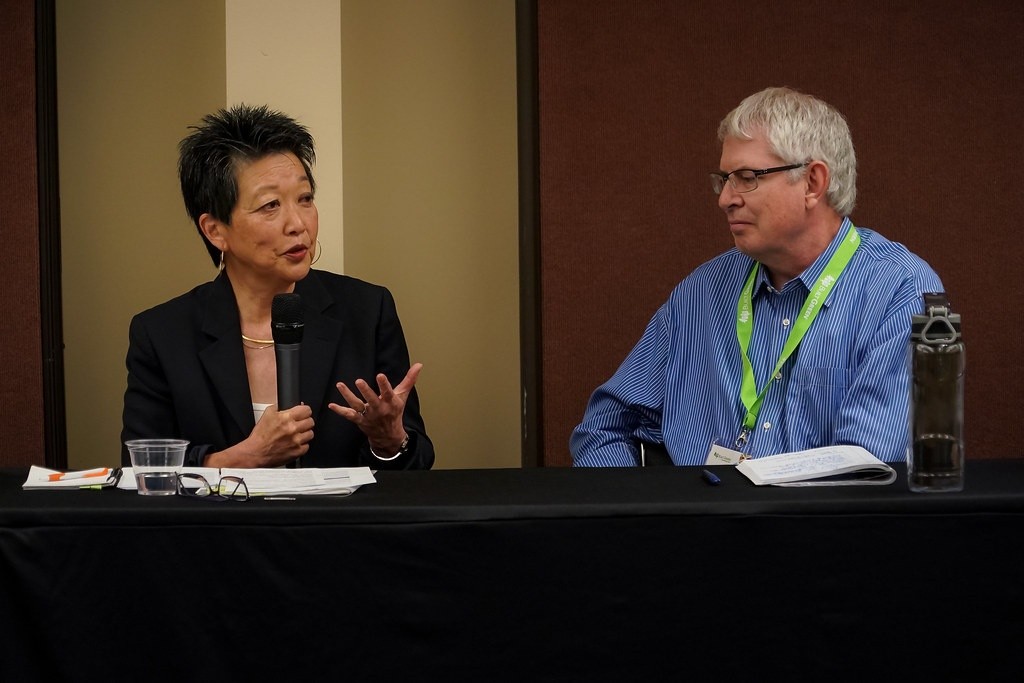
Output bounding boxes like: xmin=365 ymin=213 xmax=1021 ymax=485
xmin=357 ymin=408 xmax=368 ymax=417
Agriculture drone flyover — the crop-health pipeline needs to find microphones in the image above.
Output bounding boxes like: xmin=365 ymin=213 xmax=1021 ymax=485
xmin=270 ymin=293 xmax=306 ymax=413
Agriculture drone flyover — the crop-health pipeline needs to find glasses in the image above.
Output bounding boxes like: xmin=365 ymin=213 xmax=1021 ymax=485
xmin=708 ymin=163 xmax=810 ymax=194
xmin=174 ymin=471 xmax=251 ymax=502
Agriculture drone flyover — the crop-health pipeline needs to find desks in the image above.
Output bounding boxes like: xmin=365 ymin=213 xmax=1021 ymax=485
xmin=0 ymin=463 xmax=1024 ymax=683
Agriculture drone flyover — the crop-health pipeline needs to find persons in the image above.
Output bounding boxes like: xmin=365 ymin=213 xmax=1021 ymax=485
xmin=568 ymin=89 xmax=945 ymax=467
xmin=120 ymin=102 xmax=435 ymax=470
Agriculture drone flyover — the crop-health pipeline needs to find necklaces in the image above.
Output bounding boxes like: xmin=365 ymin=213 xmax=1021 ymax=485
xmin=242 ymin=335 xmax=275 ymax=350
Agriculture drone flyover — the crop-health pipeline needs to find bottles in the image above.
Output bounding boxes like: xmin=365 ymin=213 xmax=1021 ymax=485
xmin=912 ymin=290 xmax=965 ymax=494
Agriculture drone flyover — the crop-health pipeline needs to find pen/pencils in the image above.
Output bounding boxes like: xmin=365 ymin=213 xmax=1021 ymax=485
xmin=39 ymin=467 xmax=108 ymax=482
xmin=704 ymin=468 xmax=721 ymax=486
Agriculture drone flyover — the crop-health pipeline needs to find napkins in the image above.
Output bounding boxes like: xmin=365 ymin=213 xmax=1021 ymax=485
xmin=117 ymin=467 xmax=220 ymax=490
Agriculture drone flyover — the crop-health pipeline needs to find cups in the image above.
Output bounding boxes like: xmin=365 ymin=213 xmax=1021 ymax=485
xmin=126 ymin=439 xmax=190 ymax=495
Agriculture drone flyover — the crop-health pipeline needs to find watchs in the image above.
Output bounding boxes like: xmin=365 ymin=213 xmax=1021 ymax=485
xmin=370 ymin=433 xmax=409 ymax=461
